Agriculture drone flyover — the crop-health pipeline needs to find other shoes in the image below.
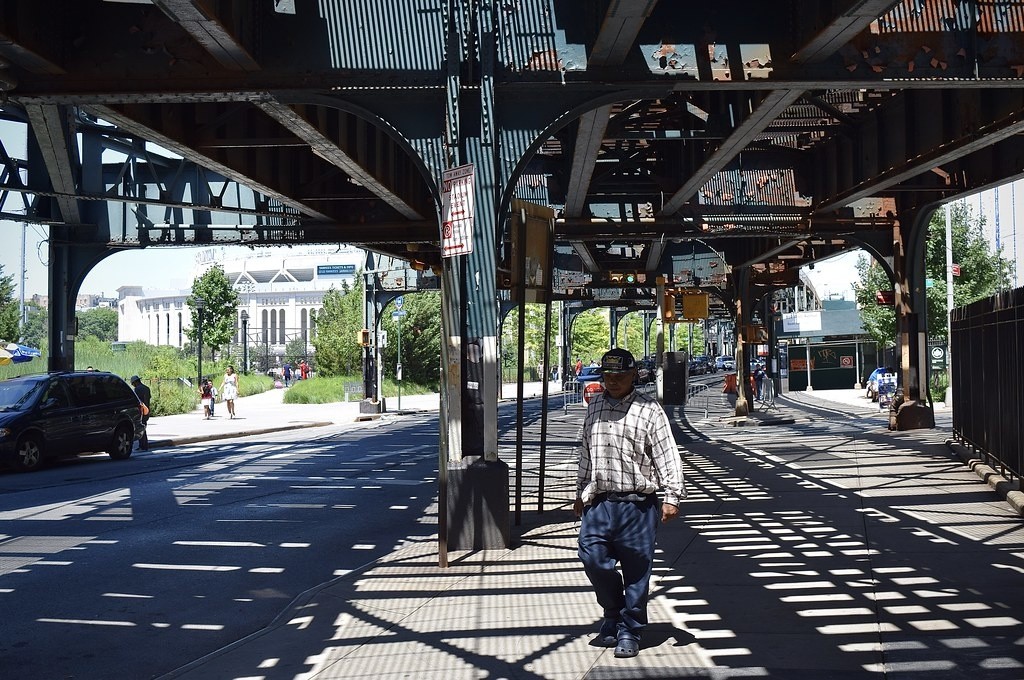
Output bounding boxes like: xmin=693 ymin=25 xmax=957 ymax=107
xmin=133 ymin=448 xmax=147 ymax=452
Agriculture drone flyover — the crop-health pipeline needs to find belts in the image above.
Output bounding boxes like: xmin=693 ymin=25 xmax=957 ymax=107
xmin=602 ymin=491 xmax=647 ymax=502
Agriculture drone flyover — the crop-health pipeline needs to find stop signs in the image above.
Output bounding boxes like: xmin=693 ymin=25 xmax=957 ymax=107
xmin=583 ymin=380 xmax=605 ymax=407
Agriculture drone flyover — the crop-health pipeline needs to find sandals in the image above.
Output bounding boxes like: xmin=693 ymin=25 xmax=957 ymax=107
xmin=614 ymin=634 xmax=639 ymax=657
xmin=600 ymin=608 xmax=619 ymax=646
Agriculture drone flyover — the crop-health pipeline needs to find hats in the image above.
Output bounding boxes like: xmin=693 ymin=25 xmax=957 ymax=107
xmin=595 ymin=348 xmax=635 ymax=373
xmin=131 ymin=376 xmax=142 ymax=383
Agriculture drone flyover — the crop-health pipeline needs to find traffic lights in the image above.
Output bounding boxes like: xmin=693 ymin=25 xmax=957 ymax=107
xmin=609 ymin=274 xmax=637 ymax=284
xmin=357 ymin=329 xmax=370 ymax=346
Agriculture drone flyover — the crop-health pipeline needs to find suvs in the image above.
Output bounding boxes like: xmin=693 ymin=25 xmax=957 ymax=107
xmin=0 ymin=370 xmax=147 ymax=473
xmin=696 ymin=355 xmax=717 ymax=374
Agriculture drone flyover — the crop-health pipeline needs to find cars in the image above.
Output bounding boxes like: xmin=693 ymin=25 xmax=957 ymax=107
xmin=575 ymin=367 xmax=603 ymax=387
xmin=690 ymin=361 xmax=705 ymax=375
xmin=635 ymin=359 xmax=657 ymax=385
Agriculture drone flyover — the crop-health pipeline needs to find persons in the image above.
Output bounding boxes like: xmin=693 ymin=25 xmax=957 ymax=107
xmin=753 ymin=364 xmax=768 ymax=400
xmin=537 ymin=359 xmax=599 ymax=383
xmin=199 ymin=380 xmax=218 ymax=420
xmin=869 ymin=367 xmax=893 ymax=402
xmin=572 ymin=348 xmax=687 ymax=627
xmin=218 ymin=366 xmax=239 ymax=418
xmin=300 ymin=360 xmax=309 ymax=380
xmin=283 ymin=361 xmax=293 ymax=387
xmin=87 ymin=366 xmax=93 ymax=373
xmin=131 ymin=376 xmax=151 ymax=451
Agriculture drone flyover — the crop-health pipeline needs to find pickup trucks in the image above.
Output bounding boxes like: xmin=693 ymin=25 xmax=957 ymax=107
xmin=716 ymin=356 xmax=737 ymax=372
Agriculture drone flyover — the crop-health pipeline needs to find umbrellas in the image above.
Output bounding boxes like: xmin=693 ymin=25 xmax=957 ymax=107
xmin=0 ymin=342 xmax=41 ymax=365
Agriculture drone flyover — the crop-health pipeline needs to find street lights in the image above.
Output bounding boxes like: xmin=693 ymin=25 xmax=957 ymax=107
xmin=241 ymin=312 xmax=250 ymax=376
xmin=195 ymin=295 xmax=205 ymax=389
